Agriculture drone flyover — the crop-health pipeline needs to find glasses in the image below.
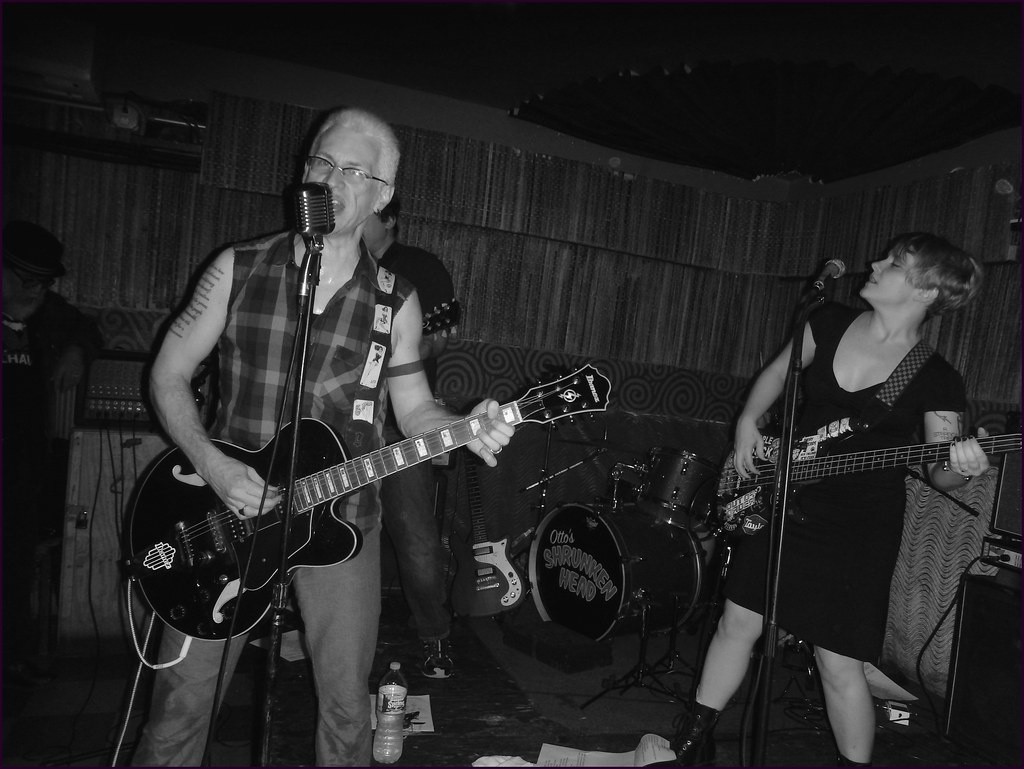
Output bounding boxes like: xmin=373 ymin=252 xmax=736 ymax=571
xmin=12 ymin=270 xmax=55 ymax=290
xmin=305 ymin=155 xmax=388 ymax=186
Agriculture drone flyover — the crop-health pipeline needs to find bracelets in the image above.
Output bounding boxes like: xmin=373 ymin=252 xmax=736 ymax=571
xmin=944 ymin=461 xmax=978 ymax=484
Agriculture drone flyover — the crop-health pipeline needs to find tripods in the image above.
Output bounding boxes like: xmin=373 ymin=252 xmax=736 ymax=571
xmin=580 ymin=596 xmax=699 ymax=713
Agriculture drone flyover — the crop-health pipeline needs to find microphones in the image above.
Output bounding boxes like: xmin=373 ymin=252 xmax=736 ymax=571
xmin=814 ymin=259 xmax=847 ymax=292
xmin=295 ymin=182 xmax=335 ymax=309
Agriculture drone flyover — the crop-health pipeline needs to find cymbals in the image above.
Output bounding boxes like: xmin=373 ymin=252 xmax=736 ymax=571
xmin=549 ymin=436 xmax=641 ymax=454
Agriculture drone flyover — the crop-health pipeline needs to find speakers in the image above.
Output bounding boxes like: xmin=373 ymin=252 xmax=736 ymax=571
xmin=941 ymin=573 xmax=1023 ymax=769
xmin=989 ymin=450 xmax=1023 ymax=538
xmin=56 ymin=428 xmax=178 ymax=645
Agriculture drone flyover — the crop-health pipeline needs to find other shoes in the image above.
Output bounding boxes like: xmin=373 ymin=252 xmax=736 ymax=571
xmin=419 ymin=635 xmax=455 ymax=679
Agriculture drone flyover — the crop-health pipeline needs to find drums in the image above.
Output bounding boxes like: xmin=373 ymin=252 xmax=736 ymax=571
xmin=528 ymin=504 xmax=702 ymax=641
xmin=635 ymin=442 xmax=718 ymax=533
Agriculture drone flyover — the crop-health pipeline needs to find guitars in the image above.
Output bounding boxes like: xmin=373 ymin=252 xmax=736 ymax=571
xmin=130 ymin=364 xmax=612 ymax=643
xmin=449 ymin=445 xmax=526 ymax=618
xmin=717 ymin=429 xmax=1022 ymax=536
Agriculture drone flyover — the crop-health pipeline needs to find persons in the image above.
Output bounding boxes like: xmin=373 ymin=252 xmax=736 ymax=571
xmin=643 ymin=230 xmax=992 ymax=768
xmin=130 ymin=108 xmax=516 ymax=769
xmin=361 ymin=195 xmax=462 ymax=682
xmin=0 ymin=220 xmax=105 ymax=687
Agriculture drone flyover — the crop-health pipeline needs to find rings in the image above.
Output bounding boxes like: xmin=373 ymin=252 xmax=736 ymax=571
xmin=492 ymin=446 xmax=503 ymax=454
xmin=959 ymin=468 xmax=967 ymax=472
xmin=72 ymin=371 xmax=79 ymax=378
xmin=237 ymin=504 xmax=248 ymax=514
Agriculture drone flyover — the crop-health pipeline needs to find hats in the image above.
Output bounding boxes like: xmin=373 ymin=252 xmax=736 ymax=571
xmin=0 ymin=219 xmax=68 ymax=276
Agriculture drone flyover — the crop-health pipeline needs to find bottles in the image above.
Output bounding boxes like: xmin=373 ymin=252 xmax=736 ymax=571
xmin=372 ymin=662 xmax=408 ymax=763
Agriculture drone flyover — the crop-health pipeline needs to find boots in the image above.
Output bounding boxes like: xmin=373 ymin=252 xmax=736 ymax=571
xmin=650 ymin=699 xmax=724 ymax=769
